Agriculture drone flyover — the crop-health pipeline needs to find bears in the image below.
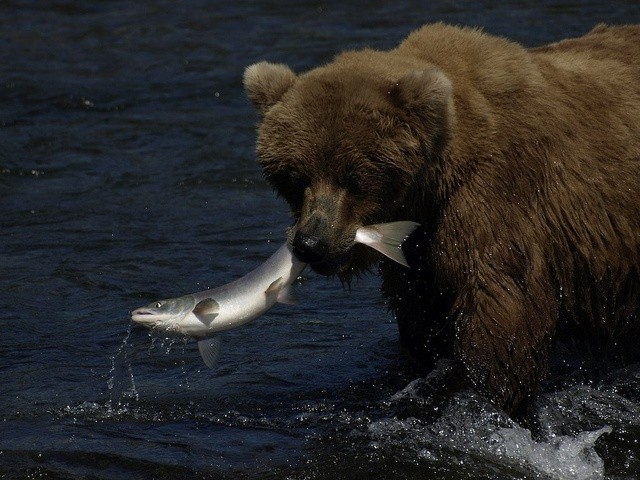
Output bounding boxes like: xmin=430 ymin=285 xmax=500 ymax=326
xmin=242 ymin=23 xmax=640 ymax=420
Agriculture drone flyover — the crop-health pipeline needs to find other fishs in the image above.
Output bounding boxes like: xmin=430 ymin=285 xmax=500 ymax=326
xmin=132 ymin=221 xmax=423 ymax=368
